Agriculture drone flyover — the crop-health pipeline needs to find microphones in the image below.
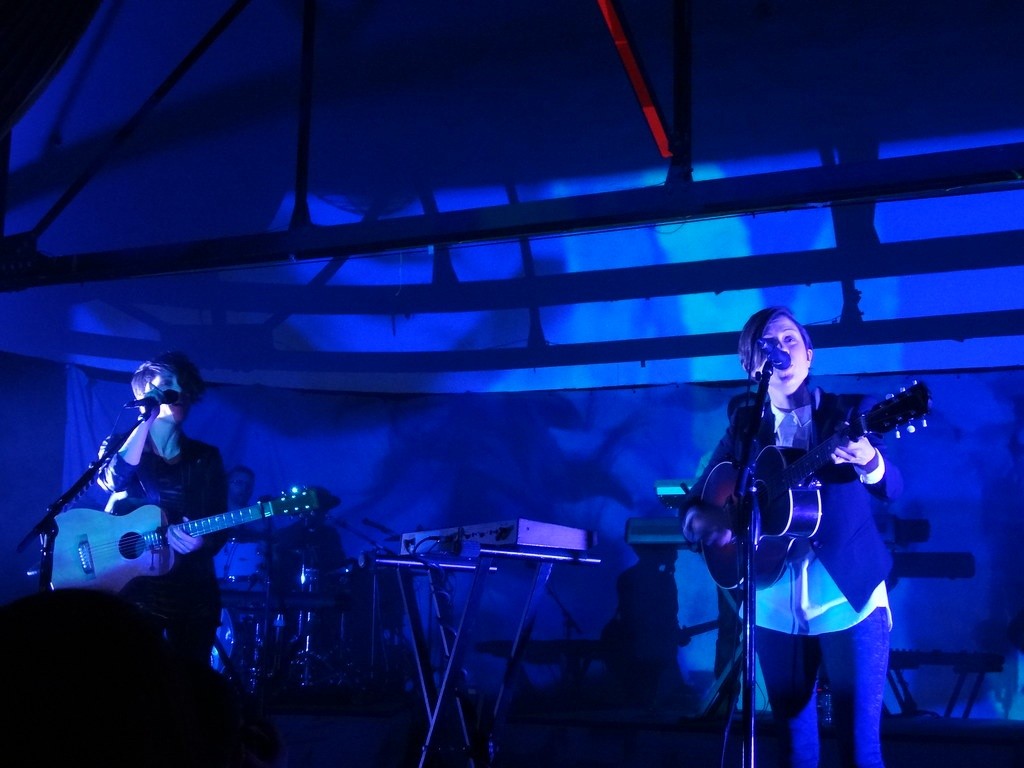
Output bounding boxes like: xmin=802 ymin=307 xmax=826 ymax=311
xmin=126 ymin=390 xmax=178 ymax=408
xmin=755 ymin=337 xmax=791 ymax=371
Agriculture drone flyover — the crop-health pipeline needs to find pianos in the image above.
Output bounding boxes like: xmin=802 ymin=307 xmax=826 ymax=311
xmin=392 ymin=519 xmax=596 ymax=561
xmin=626 ymin=514 xmax=926 ymax=550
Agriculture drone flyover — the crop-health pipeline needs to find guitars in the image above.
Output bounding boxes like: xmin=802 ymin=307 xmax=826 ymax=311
xmin=695 ymin=380 xmax=931 ymax=588
xmin=44 ymin=483 xmax=338 ymax=604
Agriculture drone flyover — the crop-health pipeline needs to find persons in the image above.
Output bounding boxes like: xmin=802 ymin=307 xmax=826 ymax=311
xmin=680 ymin=307 xmax=904 ymax=768
xmin=211 ymin=466 xmax=267 ymax=590
xmin=98 ymin=351 xmax=231 ymax=665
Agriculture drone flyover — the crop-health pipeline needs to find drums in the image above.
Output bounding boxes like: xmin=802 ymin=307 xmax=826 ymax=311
xmin=210 ymin=538 xmax=266 ymax=584
xmin=207 ymin=606 xmax=264 ymax=692
